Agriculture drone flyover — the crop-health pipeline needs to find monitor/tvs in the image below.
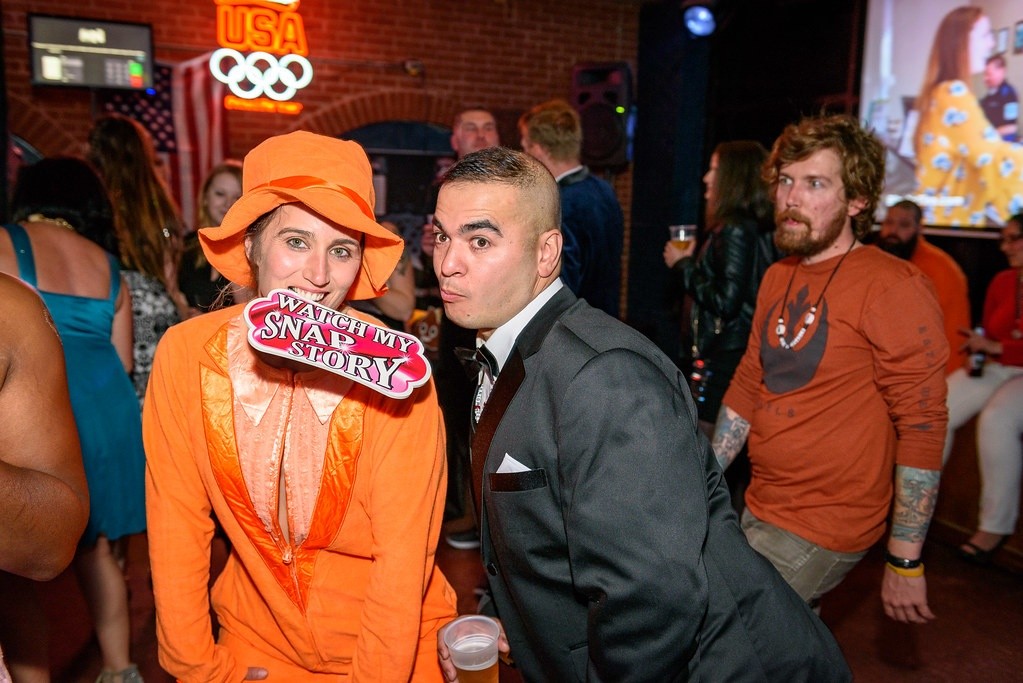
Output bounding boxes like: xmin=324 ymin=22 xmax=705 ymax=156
xmin=26 ymin=11 xmax=154 ymax=91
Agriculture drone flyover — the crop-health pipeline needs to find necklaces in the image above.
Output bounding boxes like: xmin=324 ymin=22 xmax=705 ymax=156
xmin=776 ymin=239 xmax=855 ymax=350
xmin=29 ymin=214 xmax=71 ymax=230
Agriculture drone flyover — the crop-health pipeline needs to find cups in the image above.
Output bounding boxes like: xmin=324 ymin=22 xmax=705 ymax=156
xmin=443 ymin=615 xmax=500 ymax=683
xmin=427 ymin=214 xmax=434 ymax=223
xmin=670 ymin=225 xmax=695 ymax=249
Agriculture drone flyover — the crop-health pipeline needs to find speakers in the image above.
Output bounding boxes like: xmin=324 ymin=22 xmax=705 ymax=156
xmin=569 ymin=59 xmax=636 ymax=169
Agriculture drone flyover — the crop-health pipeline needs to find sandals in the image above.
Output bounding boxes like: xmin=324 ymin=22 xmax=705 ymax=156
xmin=97 ymin=664 xmax=144 ymax=683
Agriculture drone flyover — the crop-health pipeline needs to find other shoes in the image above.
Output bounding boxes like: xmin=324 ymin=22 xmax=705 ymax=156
xmin=446 ymin=528 xmax=481 ymax=550
xmin=948 ymin=534 xmax=1010 ymax=566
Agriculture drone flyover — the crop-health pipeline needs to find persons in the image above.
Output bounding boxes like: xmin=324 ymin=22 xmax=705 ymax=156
xmin=911 ymin=5 xmax=1023 ymax=228
xmin=142 ymin=128 xmax=457 ymax=683
xmin=0 ymin=102 xmax=1023 ymax=683
xmin=981 ymin=57 xmax=1019 ymax=141
xmin=434 ymin=150 xmax=844 ymax=682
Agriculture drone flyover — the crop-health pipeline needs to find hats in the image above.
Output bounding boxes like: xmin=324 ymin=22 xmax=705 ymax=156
xmin=198 ymin=130 xmax=405 ymax=301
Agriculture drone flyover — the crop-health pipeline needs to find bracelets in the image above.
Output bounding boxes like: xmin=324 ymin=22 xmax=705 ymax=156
xmin=887 ymin=562 xmax=923 ymax=576
xmin=886 ymin=550 xmax=920 ymax=568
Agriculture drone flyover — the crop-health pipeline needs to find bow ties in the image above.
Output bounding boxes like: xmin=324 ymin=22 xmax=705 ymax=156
xmin=455 ymin=347 xmax=499 ymax=383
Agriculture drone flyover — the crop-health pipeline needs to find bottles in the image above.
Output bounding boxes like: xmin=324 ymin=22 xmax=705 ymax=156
xmin=967 ymin=326 xmax=985 ymax=377
